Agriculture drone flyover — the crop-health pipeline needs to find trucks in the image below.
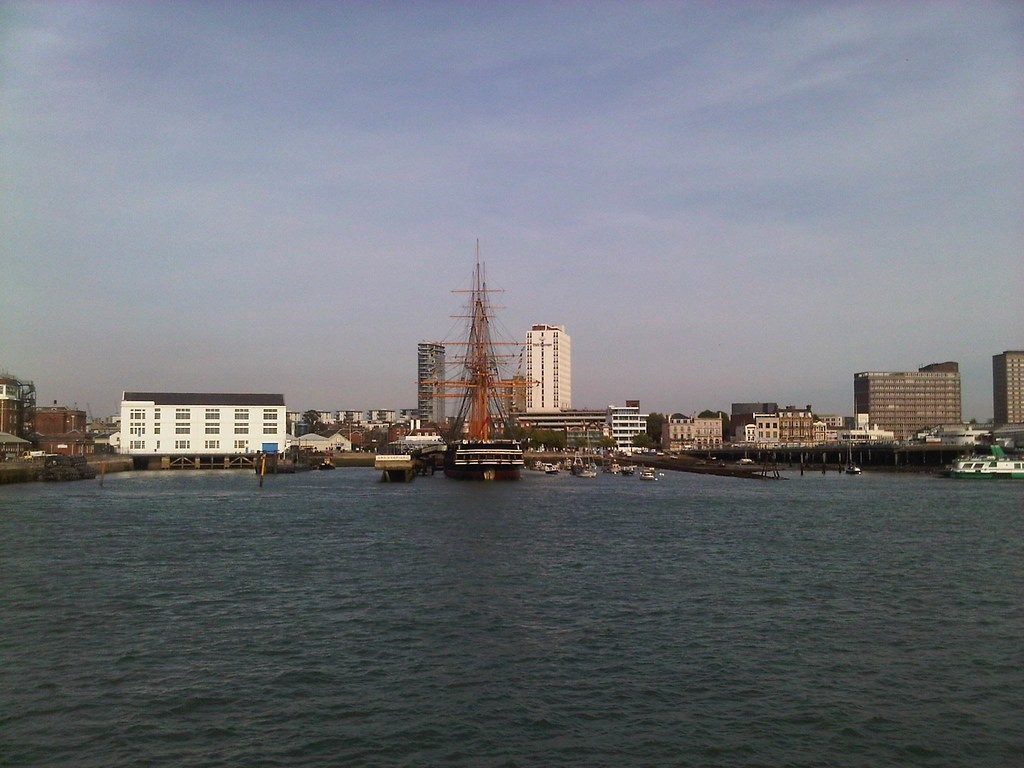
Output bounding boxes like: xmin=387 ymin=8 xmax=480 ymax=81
xmin=736 ymin=459 xmax=754 ymax=465
xmin=19 ymin=450 xmax=45 ymax=462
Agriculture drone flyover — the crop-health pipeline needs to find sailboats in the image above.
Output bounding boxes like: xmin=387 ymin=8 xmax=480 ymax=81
xmin=413 ymin=237 xmax=538 ymax=483
xmin=576 ymin=428 xmax=597 ymax=478
xmin=845 ymin=425 xmax=862 ymax=475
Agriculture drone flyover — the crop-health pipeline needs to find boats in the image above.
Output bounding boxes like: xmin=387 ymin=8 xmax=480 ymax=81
xmin=319 ymin=458 xmax=336 ymax=469
xmin=639 ymin=470 xmax=656 ymax=480
xmin=601 ymin=462 xmax=621 ymax=473
xmin=530 ymin=457 xmax=597 ymax=475
xmin=621 ymin=467 xmax=636 ymax=475
xmin=940 ymin=435 xmax=1024 ymax=480
